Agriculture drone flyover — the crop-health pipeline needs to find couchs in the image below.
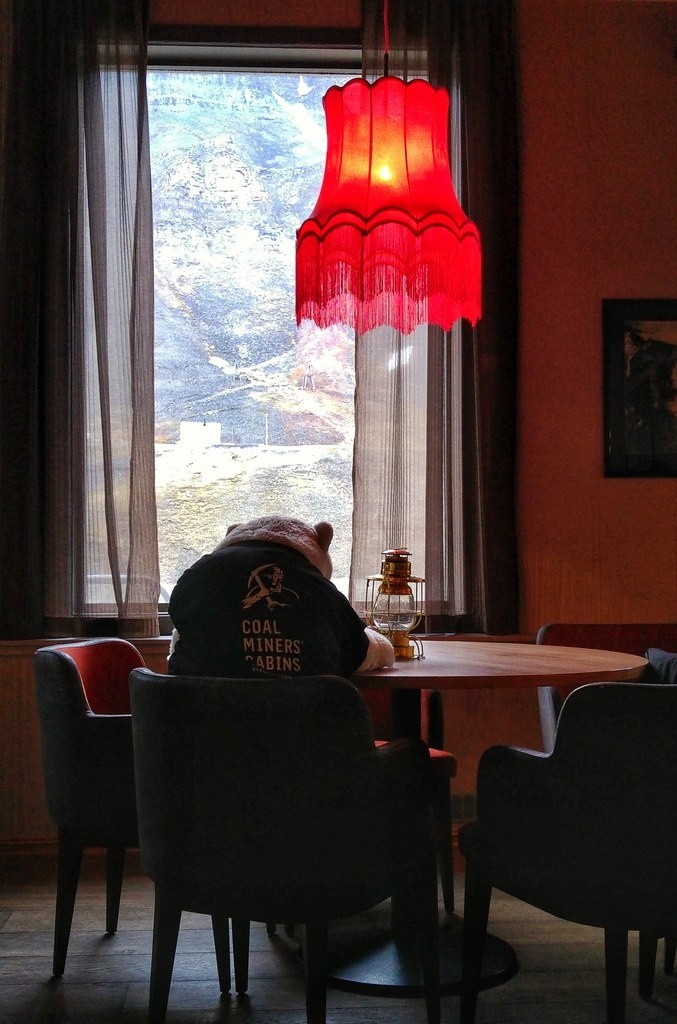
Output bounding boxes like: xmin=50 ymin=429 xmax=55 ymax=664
xmin=537 ymin=624 xmax=677 ymax=754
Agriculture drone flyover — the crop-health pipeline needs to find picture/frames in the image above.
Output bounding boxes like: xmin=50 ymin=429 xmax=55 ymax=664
xmin=602 ymin=298 xmax=677 ymax=479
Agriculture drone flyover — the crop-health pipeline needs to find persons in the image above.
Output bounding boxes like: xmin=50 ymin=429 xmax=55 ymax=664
xmin=625 ymin=332 xmax=664 ymax=446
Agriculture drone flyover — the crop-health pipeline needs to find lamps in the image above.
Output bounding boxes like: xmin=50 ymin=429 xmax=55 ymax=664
xmin=294 ymin=0 xmax=481 ymax=334
xmin=363 ymin=547 xmax=427 ymax=660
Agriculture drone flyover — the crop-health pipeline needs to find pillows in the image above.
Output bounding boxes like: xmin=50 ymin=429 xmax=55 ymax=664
xmin=644 ymin=648 xmax=677 ymax=684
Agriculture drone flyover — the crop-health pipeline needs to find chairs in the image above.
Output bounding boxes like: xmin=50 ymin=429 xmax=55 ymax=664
xmin=129 ymin=667 xmax=442 ymax=1024
xmin=34 ymin=638 xmax=231 ymax=996
xmin=457 ymin=682 xmax=677 ymax=1024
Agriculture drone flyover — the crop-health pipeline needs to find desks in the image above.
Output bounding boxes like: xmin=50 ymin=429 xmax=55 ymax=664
xmin=298 ymin=641 xmax=648 ymax=1000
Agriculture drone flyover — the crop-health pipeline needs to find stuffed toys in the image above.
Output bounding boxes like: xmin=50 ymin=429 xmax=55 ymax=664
xmin=167 ymin=516 xmax=395 ymax=680
